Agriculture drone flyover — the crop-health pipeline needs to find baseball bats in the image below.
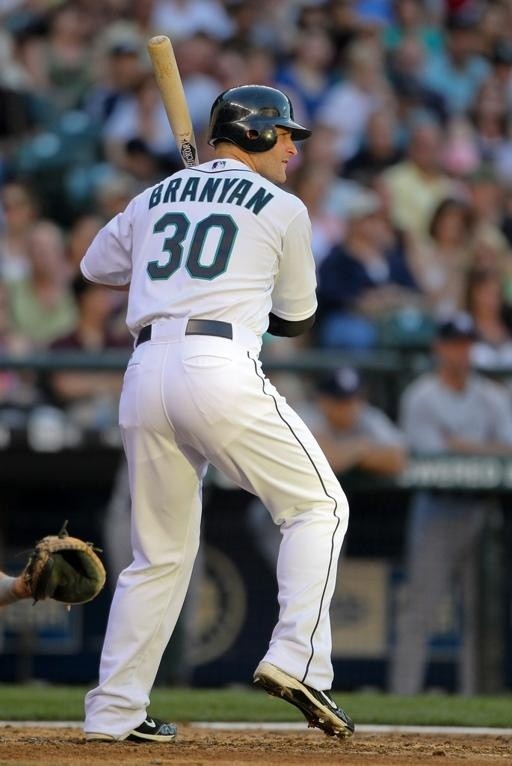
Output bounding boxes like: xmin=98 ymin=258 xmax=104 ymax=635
xmin=148 ymin=36 xmax=201 ymax=167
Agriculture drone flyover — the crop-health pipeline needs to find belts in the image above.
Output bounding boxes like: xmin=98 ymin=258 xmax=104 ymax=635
xmin=134 ymin=317 xmax=231 ymax=345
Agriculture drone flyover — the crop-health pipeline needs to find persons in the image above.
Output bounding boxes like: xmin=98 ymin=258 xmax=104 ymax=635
xmin=387 ymin=304 xmax=510 ymax=700
xmin=0 ymin=0 xmax=511 ymax=492
xmin=0 ymin=528 xmax=107 ymax=609
xmin=70 ymin=82 xmax=357 ymax=742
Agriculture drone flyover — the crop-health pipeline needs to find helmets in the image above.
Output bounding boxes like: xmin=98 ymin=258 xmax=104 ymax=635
xmin=206 ymin=85 xmax=312 ymax=153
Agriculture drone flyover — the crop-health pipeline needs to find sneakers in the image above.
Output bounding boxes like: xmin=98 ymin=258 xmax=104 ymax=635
xmin=252 ymin=662 xmax=355 ymax=738
xmin=85 ymin=716 xmax=177 ymax=744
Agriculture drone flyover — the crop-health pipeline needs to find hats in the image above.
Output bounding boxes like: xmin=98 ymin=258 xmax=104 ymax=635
xmin=322 ymin=367 xmax=360 ymax=397
xmin=439 ymin=312 xmax=483 ymax=343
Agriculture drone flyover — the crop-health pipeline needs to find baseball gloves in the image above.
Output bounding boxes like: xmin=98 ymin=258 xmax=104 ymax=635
xmin=21 ymin=535 xmax=106 ymax=604
